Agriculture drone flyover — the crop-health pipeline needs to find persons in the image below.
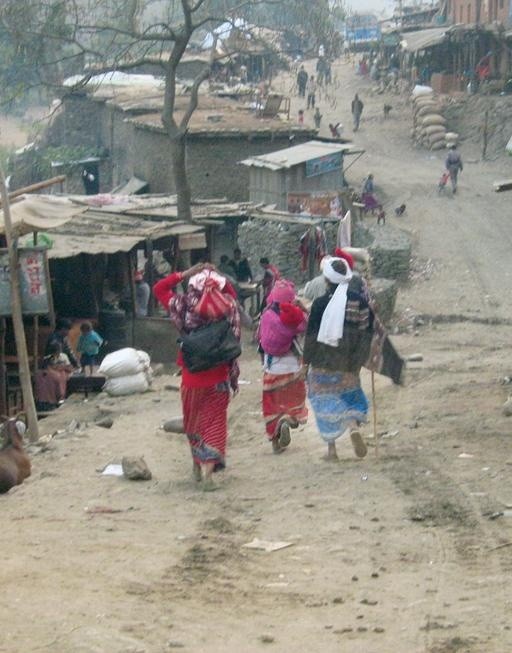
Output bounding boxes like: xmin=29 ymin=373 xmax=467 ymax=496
xmin=437 ymin=171 xmax=450 ymax=193
xmin=294 ymin=255 xmax=375 ymax=464
xmin=41 ymin=316 xmax=104 ymax=409
xmin=362 ymin=173 xmax=375 ymax=214
xmin=444 ymin=145 xmax=464 ymax=193
xmin=238 ymin=43 xmax=364 ymax=138
xmin=143 ymin=247 xmax=280 ymax=318
xmin=152 ymin=262 xmax=244 ymax=492
xmin=376 ymin=204 xmax=386 ymax=224
xmin=303 ymin=248 xmax=370 ymax=303
xmin=256 ymin=279 xmax=310 ymax=455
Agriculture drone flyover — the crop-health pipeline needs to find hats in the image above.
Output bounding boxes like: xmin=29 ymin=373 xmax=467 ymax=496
xmin=189 ymin=269 xmax=226 ymax=291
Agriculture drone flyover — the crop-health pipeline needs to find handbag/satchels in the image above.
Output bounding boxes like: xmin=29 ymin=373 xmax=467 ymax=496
xmin=177 ymin=319 xmax=240 ymax=372
xmin=194 ymin=268 xmax=232 ymax=321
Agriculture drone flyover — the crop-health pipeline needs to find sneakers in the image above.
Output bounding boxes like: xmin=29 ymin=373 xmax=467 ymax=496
xmin=350 ymin=431 xmax=367 ymax=457
xmin=279 ymin=424 xmax=291 ymax=446
